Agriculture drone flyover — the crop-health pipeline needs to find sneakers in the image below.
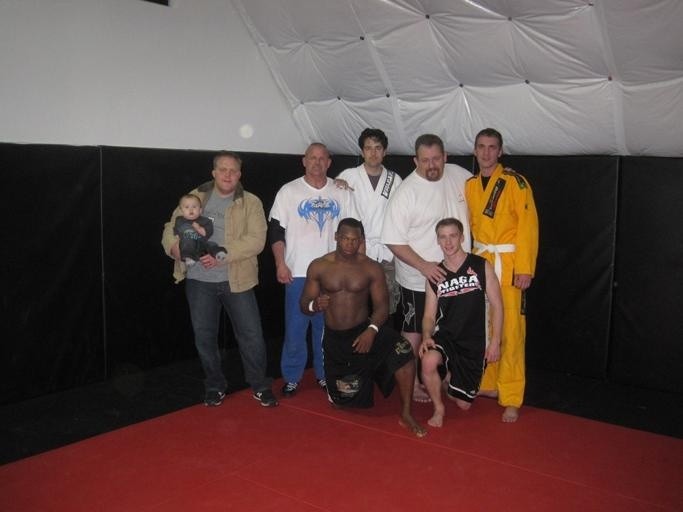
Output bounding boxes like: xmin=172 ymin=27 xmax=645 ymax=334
xmin=204 ymin=388 xmax=224 ymax=406
xmin=282 ymin=383 xmax=300 ymax=396
xmin=318 ymin=377 xmax=326 ymax=387
xmin=255 ymin=389 xmax=277 ymax=407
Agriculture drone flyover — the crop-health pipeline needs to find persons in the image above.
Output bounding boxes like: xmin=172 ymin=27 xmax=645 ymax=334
xmin=380 ymin=133 xmax=475 ymax=403
xmin=418 ymin=217 xmax=504 ymax=428
xmin=465 ymin=128 xmax=539 ymax=424
xmin=267 ymin=142 xmax=361 ymax=397
xmin=173 ymin=194 xmax=227 ymax=267
xmin=332 ymin=127 xmax=403 ymax=316
xmin=159 ymin=151 xmax=279 ymax=407
xmin=299 ymin=217 xmax=428 ymax=440
